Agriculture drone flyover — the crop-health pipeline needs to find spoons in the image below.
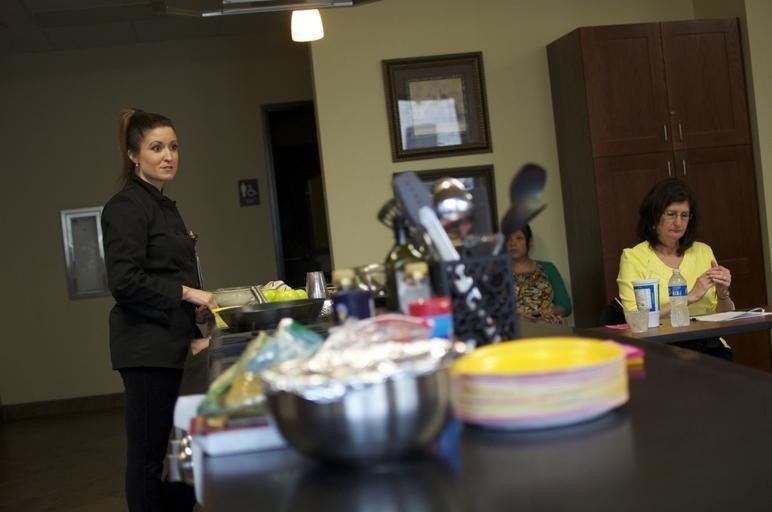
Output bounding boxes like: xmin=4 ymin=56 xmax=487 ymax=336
xmin=433 ymin=175 xmax=475 ymax=247
xmin=493 ymin=163 xmax=547 ymax=254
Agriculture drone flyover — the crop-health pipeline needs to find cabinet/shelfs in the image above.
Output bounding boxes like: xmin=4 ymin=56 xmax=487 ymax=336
xmin=546 ymin=16 xmax=770 ymax=378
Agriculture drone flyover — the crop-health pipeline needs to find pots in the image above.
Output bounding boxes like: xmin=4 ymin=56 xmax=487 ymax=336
xmin=216 ymin=294 xmax=326 ymax=331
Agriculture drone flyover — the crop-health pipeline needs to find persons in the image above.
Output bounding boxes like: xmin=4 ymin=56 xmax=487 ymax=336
xmin=616 ymin=177 xmax=736 ymax=321
xmin=100 ymin=107 xmax=221 ymax=512
xmin=505 ymin=224 xmax=573 ymax=328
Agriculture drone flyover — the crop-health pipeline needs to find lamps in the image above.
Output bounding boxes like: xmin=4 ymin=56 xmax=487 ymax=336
xmin=290 ymin=8 xmax=324 ymax=41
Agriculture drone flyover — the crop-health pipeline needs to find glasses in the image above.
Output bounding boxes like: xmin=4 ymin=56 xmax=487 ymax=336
xmin=662 ymin=209 xmax=694 ymax=221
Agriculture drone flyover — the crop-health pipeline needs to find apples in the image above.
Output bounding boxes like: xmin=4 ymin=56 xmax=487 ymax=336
xmin=262 ymin=290 xmax=276 ymax=301
xmin=275 ymin=291 xmax=299 ymax=301
xmin=295 ymin=290 xmax=307 ymax=300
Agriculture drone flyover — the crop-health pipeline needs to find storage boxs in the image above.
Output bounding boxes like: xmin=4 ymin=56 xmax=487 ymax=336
xmin=427 ymin=251 xmax=520 ymax=348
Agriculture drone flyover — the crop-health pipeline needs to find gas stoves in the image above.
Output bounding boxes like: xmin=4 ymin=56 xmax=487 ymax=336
xmin=208 ymin=324 xmax=333 ymax=345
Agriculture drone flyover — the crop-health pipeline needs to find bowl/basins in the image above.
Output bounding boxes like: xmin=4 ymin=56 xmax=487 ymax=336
xmin=260 ymin=349 xmax=449 ymax=466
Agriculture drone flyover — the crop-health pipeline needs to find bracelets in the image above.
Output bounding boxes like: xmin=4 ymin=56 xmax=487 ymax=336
xmin=715 ymin=289 xmax=730 ymax=302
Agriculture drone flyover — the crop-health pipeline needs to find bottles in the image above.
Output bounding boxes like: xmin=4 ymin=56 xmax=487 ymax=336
xmin=668 ymin=269 xmax=690 ymax=327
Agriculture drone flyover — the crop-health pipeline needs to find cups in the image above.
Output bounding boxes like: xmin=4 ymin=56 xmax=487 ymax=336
xmin=396 ymin=269 xmax=432 ymax=315
xmin=624 ymin=308 xmax=649 ymax=333
xmin=631 ymin=278 xmax=661 ymax=326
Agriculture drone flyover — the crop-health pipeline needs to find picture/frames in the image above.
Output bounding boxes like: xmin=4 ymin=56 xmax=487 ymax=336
xmin=380 ymin=51 xmax=493 ymax=163
xmin=392 ymin=165 xmax=499 ymax=245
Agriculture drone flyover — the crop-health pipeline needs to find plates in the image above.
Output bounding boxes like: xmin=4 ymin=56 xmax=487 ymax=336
xmin=449 ymin=339 xmax=630 ymax=429
